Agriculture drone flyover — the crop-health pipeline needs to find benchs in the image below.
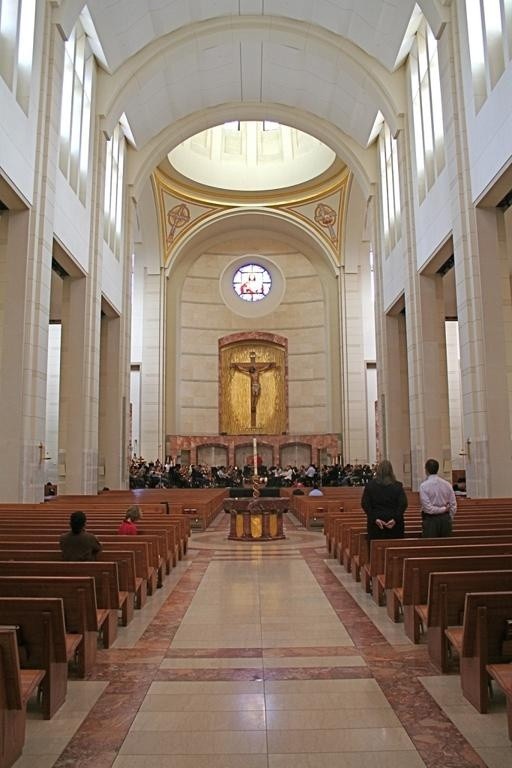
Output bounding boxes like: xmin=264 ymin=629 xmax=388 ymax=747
xmin=0 ymin=487 xmax=230 ymax=768
xmin=278 ymin=485 xmax=512 ymax=743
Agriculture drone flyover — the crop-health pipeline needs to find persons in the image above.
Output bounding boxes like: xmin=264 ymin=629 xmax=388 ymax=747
xmin=130 ymin=453 xmax=376 ymax=489
xmin=117 ymin=505 xmax=144 ymax=535
xmin=293 ymin=484 xmax=307 ymax=496
xmin=241 ymin=272 xmax=264 ymax=295
xmin=363 ymin=459 xmax=410 ymax=564
xmin=418 ymin=457 xmax=459 ymax=539
xmin=451 ymin=475 xmax=466 ymax=494
xmin=231 ymin=363 xmax=275 ymax=409
xmin=45 ymin=480 xmax=57 ymax=496
xmin=308 ymin=484 xmax=325 ymax=496
xmin=59 ymin=511 xmax=102 ymax=561
